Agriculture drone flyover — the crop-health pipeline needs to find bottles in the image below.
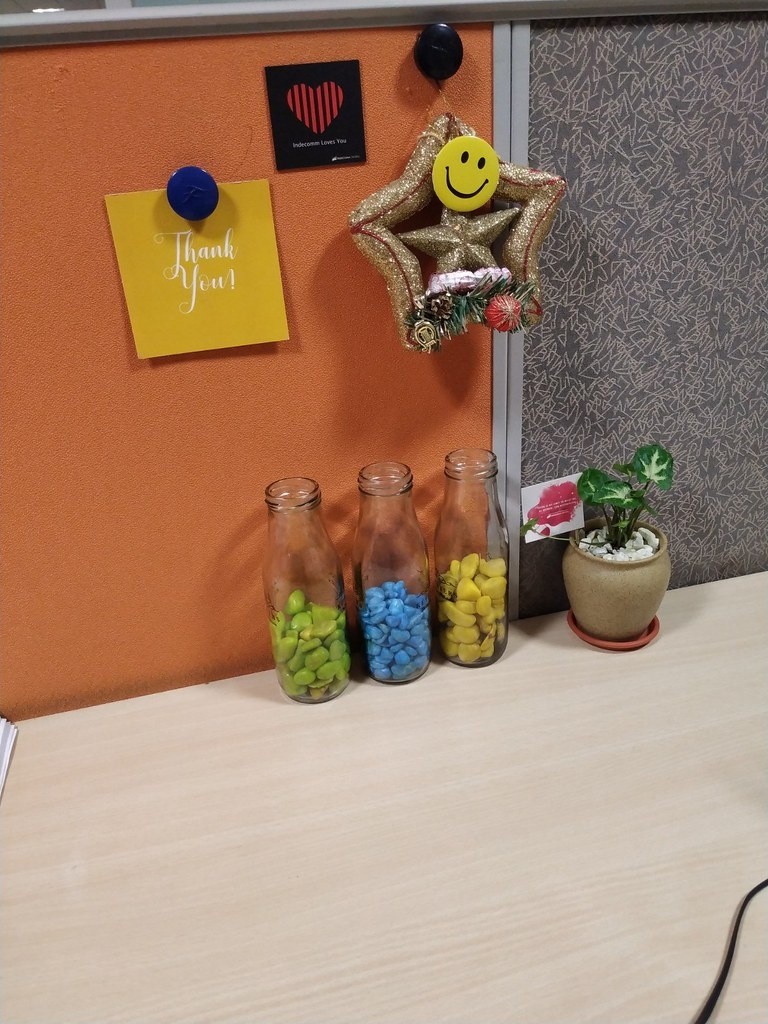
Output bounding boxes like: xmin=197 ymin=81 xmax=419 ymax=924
xmin=261 ymin=475 xmax=354 ymax=706
xmin=355 ymin=460 xmax=432 ymax=685
xmin=432 ymin=446 xmax=511 ymax=669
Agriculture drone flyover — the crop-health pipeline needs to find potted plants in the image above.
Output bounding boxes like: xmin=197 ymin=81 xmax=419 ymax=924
xmin=520 ymin=444 xmax=674 ymax=641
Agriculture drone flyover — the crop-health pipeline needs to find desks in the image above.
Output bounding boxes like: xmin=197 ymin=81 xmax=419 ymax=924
xmin=1 ymin=569 xmax=768 ymax=1024
